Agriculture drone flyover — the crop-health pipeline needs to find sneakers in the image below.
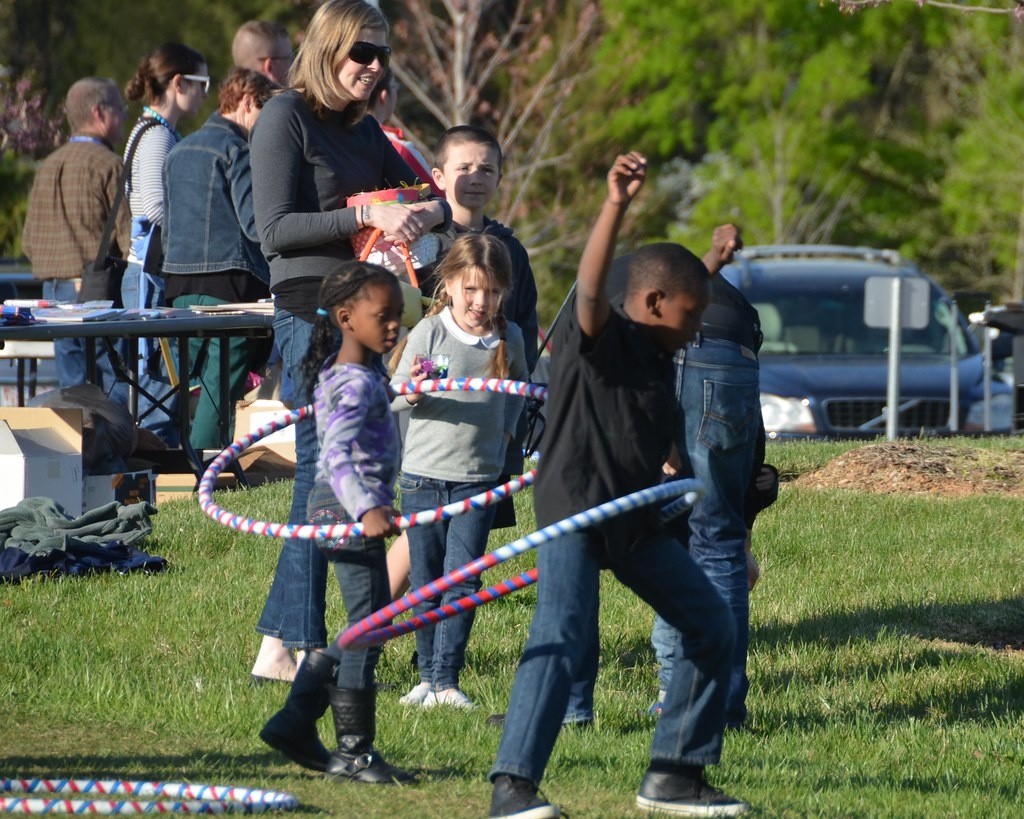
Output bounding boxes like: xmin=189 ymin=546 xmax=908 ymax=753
xmin=489 ymin=773 xmax=560 ymax=819
xmin=635 ymin=769 xmax=750 ymax=818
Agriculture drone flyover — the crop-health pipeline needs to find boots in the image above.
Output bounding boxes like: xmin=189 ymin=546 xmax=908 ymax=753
xmin=258 ymin=651 xmax=340 ymax=774
xmin=326 ymin=684 xmax=413 ymax=783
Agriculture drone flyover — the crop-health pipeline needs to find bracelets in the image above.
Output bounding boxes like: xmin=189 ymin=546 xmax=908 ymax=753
xmin=361 ymin=205 xmax=368 ymax=228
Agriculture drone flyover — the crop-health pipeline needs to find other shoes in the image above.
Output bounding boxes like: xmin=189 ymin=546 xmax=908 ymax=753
xmin=252 ymin=636 xmax=305 ymax=681
xmin=644 ymin=701 xmax=664 ymax=718
xmin=724 ymin=725 xmax=770 ymax=739
xmin=400 ymin=685 xmax=431 ymax=711
xmin=486 ymin=713 xmax=593 ymax=732
xmin=423 ymin=688 xmax=473 ymax=709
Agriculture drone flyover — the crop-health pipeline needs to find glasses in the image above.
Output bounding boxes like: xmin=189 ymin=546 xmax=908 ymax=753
xmin=258 ymin=54 xmax=292 ymax=64
xmin=170 ymin=75 xmax=210 ymax=95
xmin=98 ymin=101 xmax=129 ymax=112
xmin=389 ymin=82 xmax=400 ymax=90
xmin=336 ymin=42 xmax=391 ymax=68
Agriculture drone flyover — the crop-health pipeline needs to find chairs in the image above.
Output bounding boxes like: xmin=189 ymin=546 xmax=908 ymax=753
xmin=781 ymin=325 xmax=822 ymax=351
xmin=847 ymin=327 xmax=917 ymax=351
xmin=753 ymin=302 xmax=797 ymax=352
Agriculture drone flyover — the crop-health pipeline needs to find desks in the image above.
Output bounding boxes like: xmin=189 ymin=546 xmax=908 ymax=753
xmin=0 ymin=314 xmax=276 ymax=493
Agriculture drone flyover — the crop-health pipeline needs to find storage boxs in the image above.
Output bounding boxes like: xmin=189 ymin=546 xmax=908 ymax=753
xmin=0 ymin=406 xmax=82 ymax=519
xmin=82 ymin=468 xmax=152 ymax=515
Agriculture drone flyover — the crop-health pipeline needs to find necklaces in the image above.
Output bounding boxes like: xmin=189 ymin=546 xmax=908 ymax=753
xmin=143 ymin=105 xmax=183 ymax=143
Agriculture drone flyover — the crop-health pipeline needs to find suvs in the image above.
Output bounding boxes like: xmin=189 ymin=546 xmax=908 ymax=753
xmin=721 ymin=242 xmax=1014 ymax=441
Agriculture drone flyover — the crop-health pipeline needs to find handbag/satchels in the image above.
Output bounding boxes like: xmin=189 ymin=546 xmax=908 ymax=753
xmin=77 ymin=256 xmax=128 ymax=307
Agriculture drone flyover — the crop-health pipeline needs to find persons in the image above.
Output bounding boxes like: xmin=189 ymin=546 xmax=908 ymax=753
xmin=489 ymin=149 xmax=758 ymax=819
xmin=259 ymin=261 xmax=419 ymax=787
xmin=390 ymin=233 xmax=530 ymax=710
xmin=557 ymin=225 xmax=779 ymax=732
xmin=249 ymin=0 xmax=451 ymax=685
xmin=21 ymin=20 xmax=296 ymax=451
xmin=384 ymin=126 xmax=539 ymax=669
